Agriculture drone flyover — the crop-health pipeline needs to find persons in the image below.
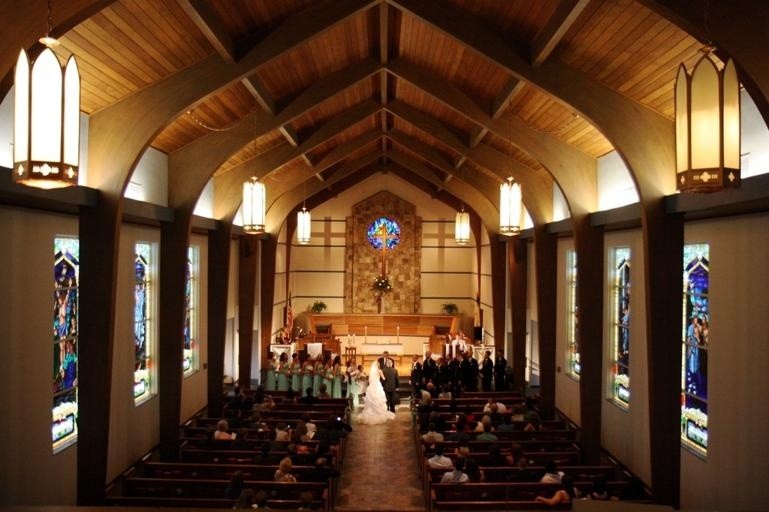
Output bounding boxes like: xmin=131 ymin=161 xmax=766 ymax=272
xmin=378 ymin=351 xmax=394 ymax=371
xmin=379 ymin=359 xmax=400 ymax=413
xmin=362 ymin=360 xmax=388 ymax=424
xmin=409 ymin=330 xmax=627 ymax=510
xmin=280 ymin=327 xmax=292 ymax=344
xmin=191 ymin=349 xmax=366 ymax=511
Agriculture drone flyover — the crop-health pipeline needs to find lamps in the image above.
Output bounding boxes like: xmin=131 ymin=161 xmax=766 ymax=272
xmin=671 ymin=51 xmax=745 ymax=192
xmin=455 ymin=167 xmax=471 ymax=247
xmin=498 ymin=100 xmax=522 ymax=236
xmin=242 ymin=114 xmax=267 ymax=235
xmin=296 ymin=160 xmax=312 ymax=246
xmin=12 ymin=0 xmax=81 ymax=192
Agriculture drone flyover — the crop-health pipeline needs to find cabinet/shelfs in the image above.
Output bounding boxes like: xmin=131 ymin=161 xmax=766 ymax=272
xmin=345 ymin=345 xmax=357 ymax=366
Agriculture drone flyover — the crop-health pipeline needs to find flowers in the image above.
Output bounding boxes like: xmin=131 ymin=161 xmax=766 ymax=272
xmin=370 ymin=274 xmax=393 ymax=296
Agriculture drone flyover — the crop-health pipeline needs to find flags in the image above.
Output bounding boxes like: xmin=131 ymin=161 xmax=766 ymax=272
xmin=472 ymin=295 xmax=483 ymax=341
xmin=285 ymin=295 xmax=293 ymax=329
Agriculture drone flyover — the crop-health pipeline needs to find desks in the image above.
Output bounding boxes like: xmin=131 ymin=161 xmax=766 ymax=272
xmin=361 ymin=341 xmax=403 ymax=366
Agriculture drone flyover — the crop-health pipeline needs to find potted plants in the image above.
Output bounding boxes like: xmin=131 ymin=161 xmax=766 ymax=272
xmin=440 ymin=303 xmax=459 ymax=315
xmin=311 ymin=300 xmax=327 ymax=313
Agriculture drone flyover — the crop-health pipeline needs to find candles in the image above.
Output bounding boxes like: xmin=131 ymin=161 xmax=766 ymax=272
xmin=397 ymin=326 xmax=401 ymax=343
xmin=364 ymin=326 xmax=367 ymax=343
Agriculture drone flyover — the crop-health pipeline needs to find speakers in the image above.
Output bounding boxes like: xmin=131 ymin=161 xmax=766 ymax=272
xmin=473 ymin=326 xmax=484 ymax=345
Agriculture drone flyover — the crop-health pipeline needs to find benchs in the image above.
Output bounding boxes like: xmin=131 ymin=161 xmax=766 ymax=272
xmin=101 ymin=392 xmax=353 ymax=512
xmin=411 ymin=383 xmax=653 ymax=512
